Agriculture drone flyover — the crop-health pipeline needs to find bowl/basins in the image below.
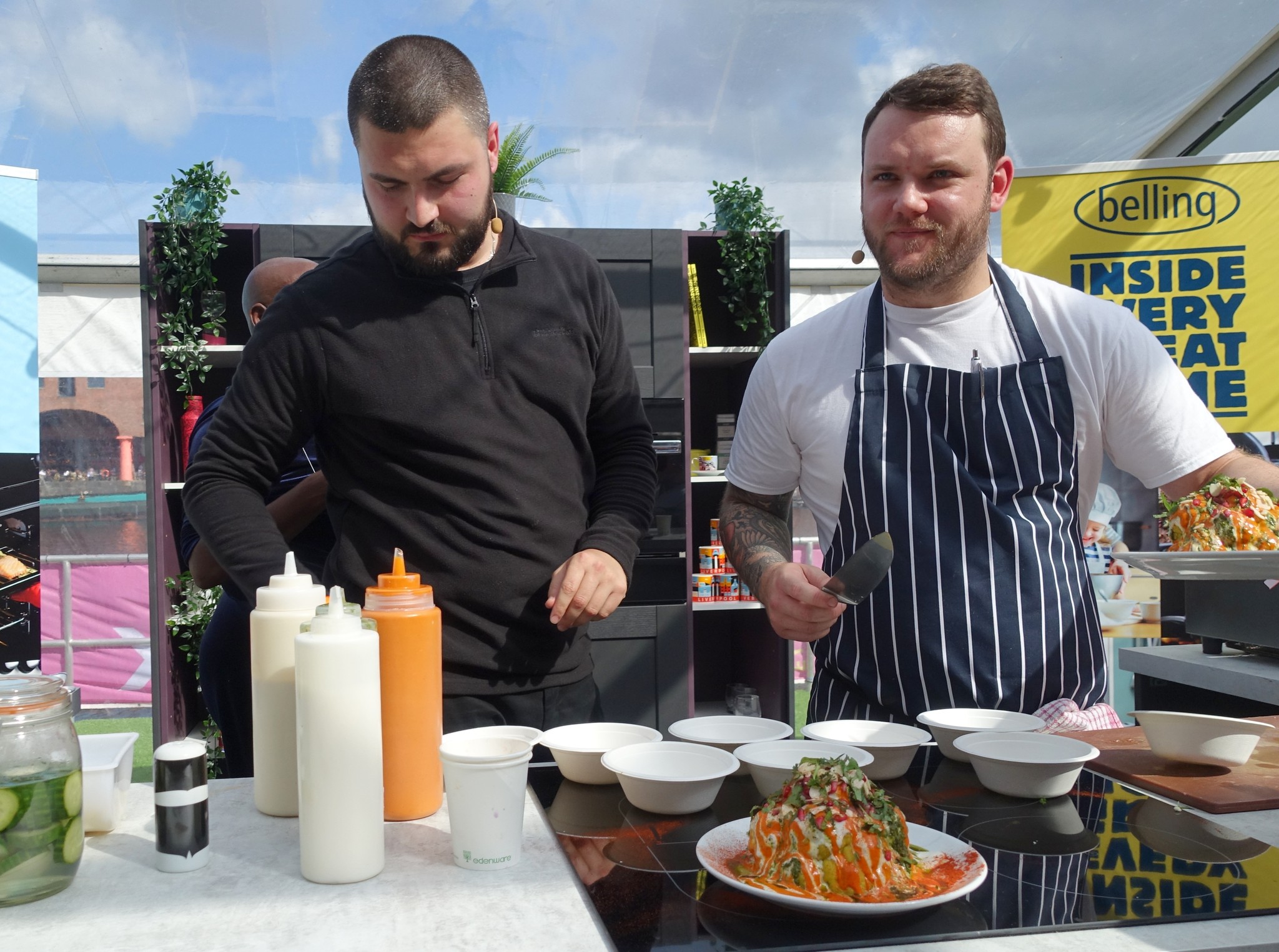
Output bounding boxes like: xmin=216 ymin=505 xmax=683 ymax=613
xmin=1126 ymin=711 xmax=1275 ymax=768
xmin=549 ymin=778 xmax=1278 ymax=875
xmin=800 ymin=719 xmax=932 ymax=780
xmin=441 ymin=725 xmax=545 ymax=751
xmin=952 ymin=731 xmax=1101 ymax=799
xmin=733 ymin=740 xmax=875 ymax=802
xmin=539 ymin=722 xmax=663 ymax=785
xmin=916 ymin=707 xmax=1047 ymax=763
xmin=667 ymin=714 xmax=794 ymax=776
xmin=918 ymin=762 xmax=1270 ymax=862
xmin=1088 ymin=573 xmax=1161 ymax=624
xmin=601 ymin=740 xmax=741 ymax=814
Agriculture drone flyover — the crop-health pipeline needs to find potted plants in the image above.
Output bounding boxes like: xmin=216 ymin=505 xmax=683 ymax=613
xmin=164 ymin=571 xmax=224 ymax=780
xmin=140 ymin=159 xmax=240 ymax=410
xmin=699 ymin=178 xmax=783 ymax=358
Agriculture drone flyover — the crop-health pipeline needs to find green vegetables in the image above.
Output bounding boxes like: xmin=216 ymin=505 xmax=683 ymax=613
xmin=1152 ymin=475 xmax=1279 ymax=551
xmin=750 ymin=753 xmax=929 ymax=885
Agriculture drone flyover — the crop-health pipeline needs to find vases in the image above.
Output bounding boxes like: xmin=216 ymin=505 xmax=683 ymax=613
xmin=181 ymin=395 xmax=204 ymax=475
xmin=201 ymin=290 xmax=227 ymax=346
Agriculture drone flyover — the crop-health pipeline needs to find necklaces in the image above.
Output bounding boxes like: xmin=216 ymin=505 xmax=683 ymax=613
xmin=486 ymin=229 xmax=496 ymax=263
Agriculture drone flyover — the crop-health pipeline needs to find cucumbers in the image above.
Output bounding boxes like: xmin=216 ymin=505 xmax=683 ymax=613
xmin=0 ymin=765 xmax=84 ymax=876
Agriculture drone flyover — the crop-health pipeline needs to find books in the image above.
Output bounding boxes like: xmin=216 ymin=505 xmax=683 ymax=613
xmin=686 ymin=262 xmax=708 ymax=346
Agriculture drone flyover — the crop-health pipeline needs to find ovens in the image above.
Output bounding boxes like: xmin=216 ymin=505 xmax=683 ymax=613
xmin=611 ymin=395 xmax=690 ymax=606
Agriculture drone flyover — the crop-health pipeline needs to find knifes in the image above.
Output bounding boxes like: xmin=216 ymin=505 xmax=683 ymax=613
xmin=821 ymin=531 xmax=895 ymax=608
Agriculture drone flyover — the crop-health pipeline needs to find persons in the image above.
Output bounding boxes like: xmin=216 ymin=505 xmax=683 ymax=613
xmin=1082 ymin=483 xmax=1131 ymax=599
xmin=180 ymin=255 xmax=328 ymax=782
xmin=558 ymin=836 xmax=617 ymax=886
xmin=718 ymin=64 xmax=1279 ymax=725
xmin=182 ymin=35 xmax=654 ymax=760
xmin=906 ymin=749 xmax=1112 ymax=929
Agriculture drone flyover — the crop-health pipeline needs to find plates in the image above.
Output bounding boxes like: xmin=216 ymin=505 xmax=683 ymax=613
xmin=690 ymin=469 xmax=726 ymax=476
xmin=1109 ymin=546 xmax=1279 ymax=581
xmin=697 ymin=817 xmax=987 ymax=916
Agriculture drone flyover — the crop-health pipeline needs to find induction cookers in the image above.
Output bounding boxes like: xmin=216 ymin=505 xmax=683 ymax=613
xmin=520 ymin=722 xmax=1279 ymax=951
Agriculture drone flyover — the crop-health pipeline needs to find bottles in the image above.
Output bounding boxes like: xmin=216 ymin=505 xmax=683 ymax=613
xmin=294 ymin=584 xmax=387 ymax=884
xmin=248 ymin=551 xmax=330 ymax=817
xmin=0 ymin=673 xmax=84 ymax=907
xmin=360 ymin=547 xmax=446 ymax=821
xmin=302 ymin=586 xmax=378 ymax=633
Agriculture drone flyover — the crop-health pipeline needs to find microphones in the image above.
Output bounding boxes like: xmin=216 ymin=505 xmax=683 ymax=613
xmin=491 ymin=198 xmax=503 ymax=233
xmin=852 ymin=240 xmax=867 ymax=265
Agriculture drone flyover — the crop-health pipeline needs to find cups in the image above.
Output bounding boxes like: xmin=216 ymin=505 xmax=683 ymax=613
xmin=437 ymin=726 xmax=533 ymax=870
xmin=691 ymin=455 xmax=718 ymax=471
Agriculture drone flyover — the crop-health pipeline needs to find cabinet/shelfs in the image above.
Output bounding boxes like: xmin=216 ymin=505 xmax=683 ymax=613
xmin=137 ymin=219 xmax=794 ymax=777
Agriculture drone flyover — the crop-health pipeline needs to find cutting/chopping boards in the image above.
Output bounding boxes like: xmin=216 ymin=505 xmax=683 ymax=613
xmin=1058 ymin=706 xmax=1278 ymax=814
